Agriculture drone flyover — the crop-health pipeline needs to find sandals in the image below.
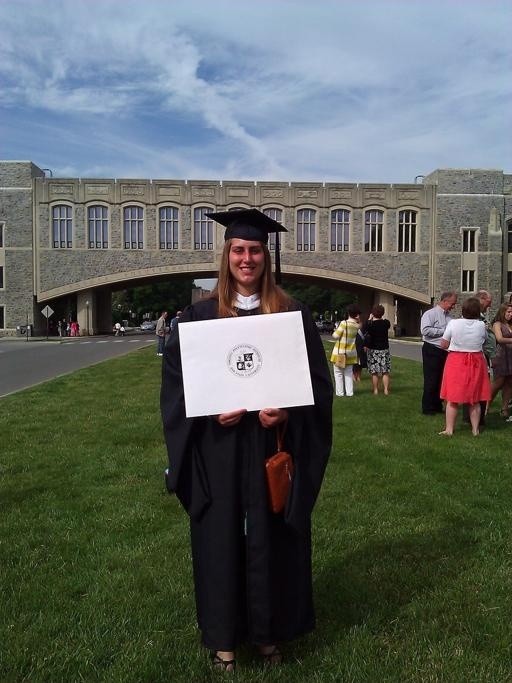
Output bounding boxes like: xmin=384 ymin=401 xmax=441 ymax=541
xmin=261 ymin=645 xmax=282 ymax=668
xmin=212 ymin=650 xmax=236 ymax=674
xmin=500 ymin=407 xmax=509 ymax=419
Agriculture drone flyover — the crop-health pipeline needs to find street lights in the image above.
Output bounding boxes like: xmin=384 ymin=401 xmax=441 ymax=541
xmin=85 ymin=300 xmax=90 ymax=335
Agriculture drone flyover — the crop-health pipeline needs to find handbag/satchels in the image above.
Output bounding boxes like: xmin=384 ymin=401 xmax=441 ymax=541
xmin=266 ymin=409 xmax=295 ymax=515
xmin=335 ymin=353 xmax=347 ymax=369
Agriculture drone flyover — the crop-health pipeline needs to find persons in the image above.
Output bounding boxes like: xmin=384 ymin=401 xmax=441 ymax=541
xmin=486 ymin=302 xmax=512 ymax=419
xmin=113 ymin=321 xmax=125 ymax=337
xmin=58 ymin=318 xmax=80 ymax=336
xmin=162 ymin=208 xmax=334 ymax=675
xmin=462 ymin=290 xmax=497 ymax=426
xmin=438 ymin=298 xmax=488 ymax=436
xmin=421 ymin=292 xmax=459 ymax=415
xmin=331 ymin=304 xmax=391 ymax=396
xmin=155 ymin=311 xmax=167 ymax=356
xmin=170 ymin=310 xmax=182 ymax=330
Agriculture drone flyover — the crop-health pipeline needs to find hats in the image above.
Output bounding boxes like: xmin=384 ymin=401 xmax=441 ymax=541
xmin=204 ymin=207 xmax=288 ymax=243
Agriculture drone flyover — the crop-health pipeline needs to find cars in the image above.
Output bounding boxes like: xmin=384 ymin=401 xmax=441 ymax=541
xmin=140 ymin=321 xmax=155 ymax=331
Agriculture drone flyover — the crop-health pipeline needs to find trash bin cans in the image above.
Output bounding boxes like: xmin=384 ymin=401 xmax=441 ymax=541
xmin=16 ymin=325 xmax=31 ymax=337
